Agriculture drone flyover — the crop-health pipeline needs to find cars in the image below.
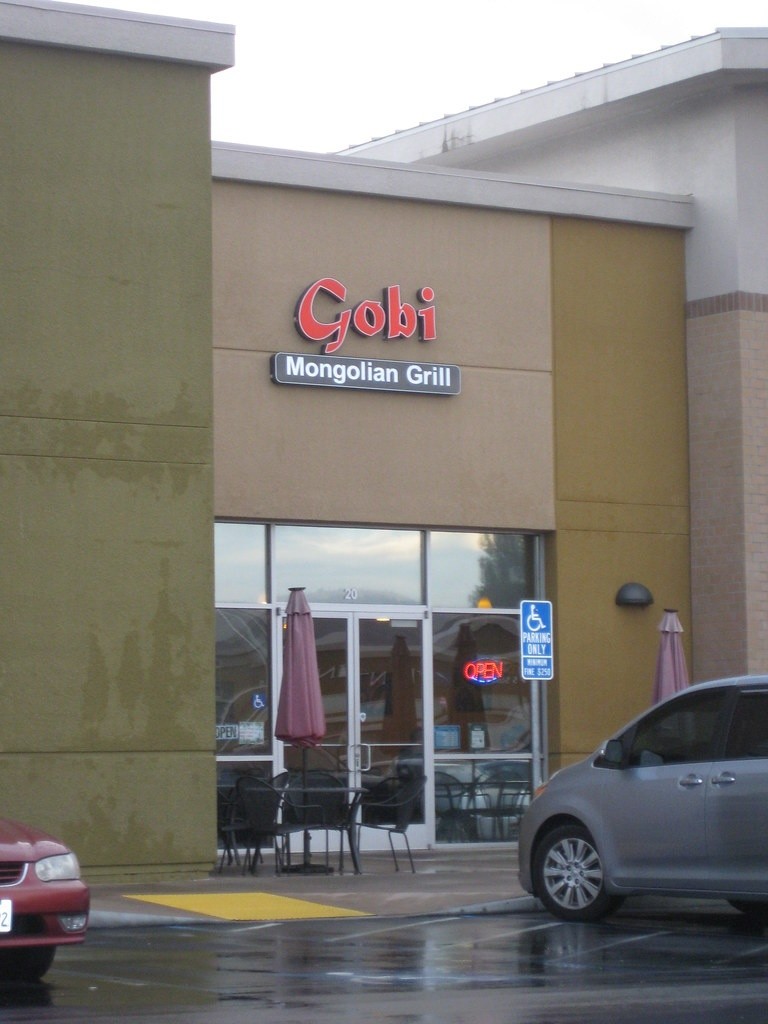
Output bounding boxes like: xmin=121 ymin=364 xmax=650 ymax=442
xmin=515 ymin=673 xmax=768 ymax=924
xmin=367 ymin=757 xmax=533 ymax=845
xmin=0 ymin=814 xmax=92 ymax=991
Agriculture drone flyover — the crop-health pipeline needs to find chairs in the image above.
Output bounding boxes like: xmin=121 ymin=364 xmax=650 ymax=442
xmin=336 ymin=772 xmax=428 ymax=875
xmin=432 ymin=757 xmax=532 ymax=842
xmin=236 ymin=776 xmax=331 ymax=877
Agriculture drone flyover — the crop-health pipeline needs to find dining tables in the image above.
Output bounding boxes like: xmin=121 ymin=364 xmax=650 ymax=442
xmin=217 ymin=784 xmax=371 ymax=877
